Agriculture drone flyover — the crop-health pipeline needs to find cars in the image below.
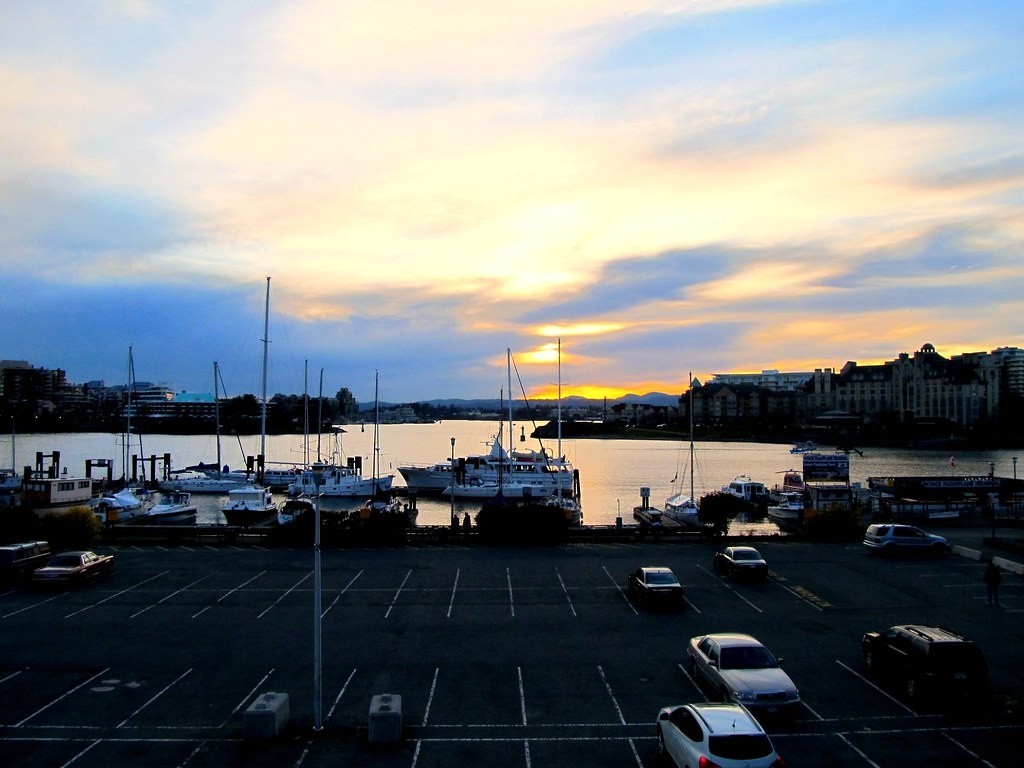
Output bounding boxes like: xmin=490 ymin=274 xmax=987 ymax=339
xmin=656 ymin=703 xmax=784 ymax=768
xmin=33 ymin=551 xmax=114 ymax=583
xmin=714 ymin=547 xmax=768 ymax=577
xmin=686 ymin=633 xmax=800 ymax=711
xmin=628 ymin=567 xmax=683 ymax=601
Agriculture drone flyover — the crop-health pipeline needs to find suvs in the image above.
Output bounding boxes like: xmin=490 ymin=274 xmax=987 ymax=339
xmin=863 ymin=625 xmax=986 ymax=700
xmin=863 ymin=524 xmax=950 ymax=555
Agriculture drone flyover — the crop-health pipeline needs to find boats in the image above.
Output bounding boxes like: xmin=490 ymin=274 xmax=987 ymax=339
xmin=789 ymin=440 xmax=820 ymax=454
xmin=722 ymin=470 xmax=893 ymax=519
xmin=10 ymin=467 xmax=317 ymax=526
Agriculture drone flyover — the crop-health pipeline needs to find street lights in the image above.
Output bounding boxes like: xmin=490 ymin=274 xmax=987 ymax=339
xmin=312 ymin=462 xmax=327 ymax=731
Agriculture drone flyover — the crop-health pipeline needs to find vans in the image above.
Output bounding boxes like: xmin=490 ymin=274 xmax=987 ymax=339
xmin=0 ymin=541 xmax=51 ymax=575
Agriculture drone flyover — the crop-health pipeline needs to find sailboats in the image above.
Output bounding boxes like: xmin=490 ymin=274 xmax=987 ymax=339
xmin=397 ymin=340 xmax=583 ymax=525
xmin=665 ymin=372 xmax=706 ymax=527
xmin=115 ymin=278 xmax=395 ymax=496
xmin=0 ymin=406 xmax=24 ymax=493
